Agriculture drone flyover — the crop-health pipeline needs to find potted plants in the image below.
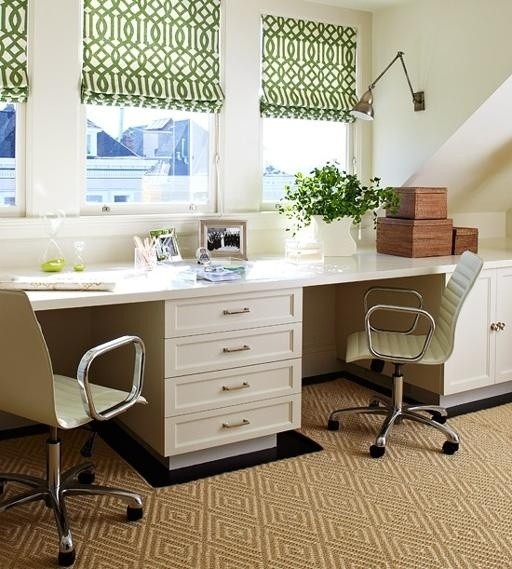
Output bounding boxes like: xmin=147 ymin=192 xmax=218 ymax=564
xmin=273 ymin=156 xmax=401 ymax=257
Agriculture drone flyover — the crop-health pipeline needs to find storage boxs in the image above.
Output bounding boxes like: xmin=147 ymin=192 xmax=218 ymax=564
xmin=372 ymin=185 xmax=480 ymax=258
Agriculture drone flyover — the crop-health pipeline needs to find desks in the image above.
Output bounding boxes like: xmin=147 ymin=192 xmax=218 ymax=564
xmin=0 ymin=247 xmax=510 ymax=472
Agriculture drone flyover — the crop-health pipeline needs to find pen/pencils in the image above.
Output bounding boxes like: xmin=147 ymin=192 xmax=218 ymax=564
xmin=133 ymin=236 xmax=160 ymax=269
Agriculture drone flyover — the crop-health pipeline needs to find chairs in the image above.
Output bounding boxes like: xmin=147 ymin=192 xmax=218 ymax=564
xmin=0 ymin=288 xmax=152 ymax=568
xmin=326 ymin=251 xmax=484 ymax=460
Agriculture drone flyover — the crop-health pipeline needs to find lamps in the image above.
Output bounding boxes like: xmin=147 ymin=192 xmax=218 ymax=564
xmin=349 ymin=49 xmax=424 ymax=124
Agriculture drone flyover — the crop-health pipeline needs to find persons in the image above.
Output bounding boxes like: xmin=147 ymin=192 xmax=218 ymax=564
xmin=208 ymin=231 xmax=240 ymax=251
xmin=200 ymin=249 xmax=208 ymax=261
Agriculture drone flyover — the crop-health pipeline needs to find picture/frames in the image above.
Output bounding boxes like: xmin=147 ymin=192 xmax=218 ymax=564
xmin=150 ymin=227 xmax=183 ymax=263
xmin=198 ymin=219 xmax=247 ymax=259
